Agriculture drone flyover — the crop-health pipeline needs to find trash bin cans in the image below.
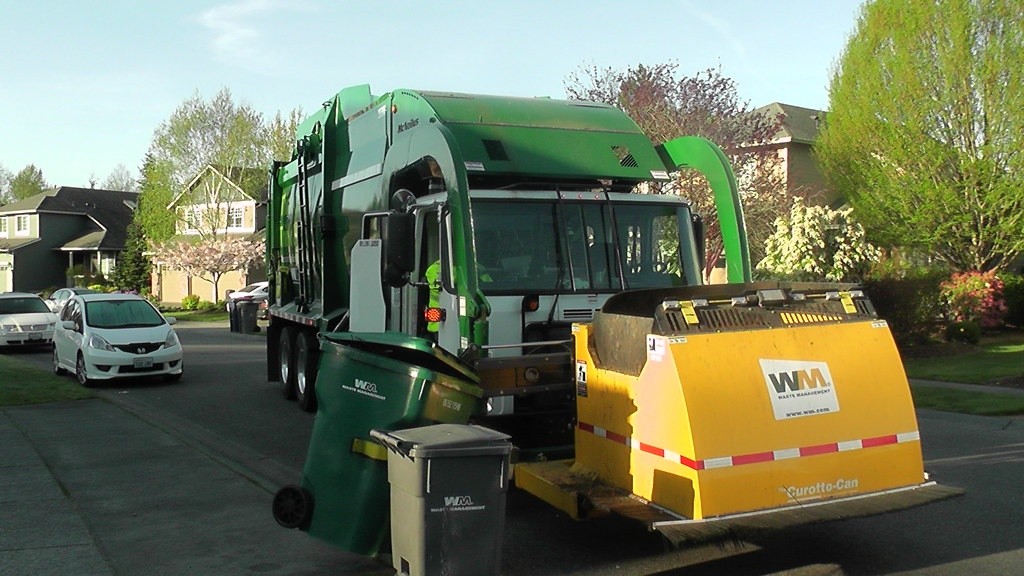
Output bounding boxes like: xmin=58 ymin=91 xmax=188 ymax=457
xmin=271 ymin=330 xmax=485 ymax=568
xmin=228 ymin=298 xmax=262 ymax=334
xmin=369 ymin=424 xmax=516 ymax=576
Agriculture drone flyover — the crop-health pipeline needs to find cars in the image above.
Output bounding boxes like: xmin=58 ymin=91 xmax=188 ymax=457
xmin=51 ymin=292 xmax=185 ymax=389
xmin=0 ymin=292 xmax=59 ymax=354
xmin=44 ymin=287 xmax=99 ymax=316
xmin=224 ymin=281 xmax=270 ymax=319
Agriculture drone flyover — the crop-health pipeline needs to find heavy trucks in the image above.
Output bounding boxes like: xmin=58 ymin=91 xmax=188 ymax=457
xmin=255 ymin=83 xmax=938 ymax=545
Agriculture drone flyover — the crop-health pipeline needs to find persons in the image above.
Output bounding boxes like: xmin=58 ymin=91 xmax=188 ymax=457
xmin=424 ymin=256 xmax=494 ymax=347
